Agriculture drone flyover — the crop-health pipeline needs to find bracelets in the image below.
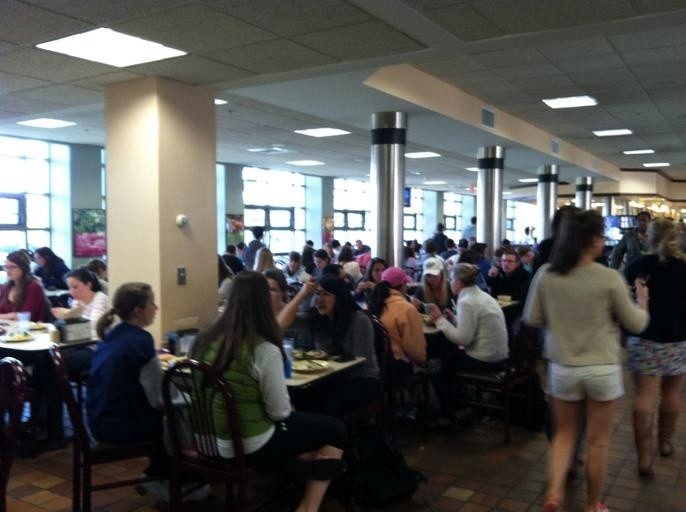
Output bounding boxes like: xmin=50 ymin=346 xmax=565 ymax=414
xmin=635 ymin=296 xmax=650 ymax=302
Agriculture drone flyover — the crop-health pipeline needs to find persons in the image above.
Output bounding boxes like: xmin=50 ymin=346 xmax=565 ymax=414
xmin=0 ymin=248 xmax=117 ymax=452
xmin=607 ymin=212 xmax=653 ymax=346
xmin=85 ymin=282 xmax=181 ymax=495
xmin=277 ymin=273 xmax=381 ymax=419
xmin=522 ymin=209 xmax=650 ymax=511
xmin=368 ymin=206 xmax=580 ymax=488
xmin=189 ymin=270 xmax=346 ymax=512
xmin=675 ymin=219 xmax=685 ymax=253
xmin=217 ymin=222 xmax=389 ymax=326
xmin=623 ymin=217 xmax=686 ymax=478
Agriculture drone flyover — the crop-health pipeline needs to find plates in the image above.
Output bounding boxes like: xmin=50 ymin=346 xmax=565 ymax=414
xmin=294 ymin=350 xmax=327 ymax=359
xmin=30 ymin=325 xmax=47 ymax=330
xmin=291 ymin=359 xmax=328 ymax=371
xmin=0 ymin=332 xmax=34 ymax=341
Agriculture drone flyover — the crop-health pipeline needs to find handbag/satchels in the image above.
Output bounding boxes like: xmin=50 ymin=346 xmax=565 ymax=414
xmin=490 ymin=369 xmax=546 ymax=432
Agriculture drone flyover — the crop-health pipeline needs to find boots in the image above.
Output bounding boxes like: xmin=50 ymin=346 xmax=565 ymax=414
xmin=634 ymin=412 xmax=655 ymax=476
xmin=659 ymin=406 xmax=679 ymax=456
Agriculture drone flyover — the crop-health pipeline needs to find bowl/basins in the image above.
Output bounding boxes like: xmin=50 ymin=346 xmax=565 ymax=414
xmin=497 ymin=295 xmax=512 ymax=303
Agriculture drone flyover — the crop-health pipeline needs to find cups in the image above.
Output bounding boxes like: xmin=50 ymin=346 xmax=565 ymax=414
xmin=17 ymin=312 xmax=31 ymax=329
xmin=282 ymin=337 xmax=294 ymax=359
xmin=166 ymin=331 xmax=178 ymax=355
xmin=423 ymin=303 xmax=435 ymax=314
xmin=284 ymin=360 xmax=291 ymax=378
xmin=184 ymin=335 xmax=196 ymax=355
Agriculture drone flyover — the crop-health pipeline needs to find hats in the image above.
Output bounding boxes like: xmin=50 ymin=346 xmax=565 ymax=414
xmin=382 ymin=266 xmax=413 ymax=287
xmin=422 ymin=258 xmax=443 ymax=276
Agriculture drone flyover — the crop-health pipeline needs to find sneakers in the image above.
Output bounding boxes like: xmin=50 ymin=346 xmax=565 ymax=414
xmin=291 ymin=459 xmax=345 ymax=480
